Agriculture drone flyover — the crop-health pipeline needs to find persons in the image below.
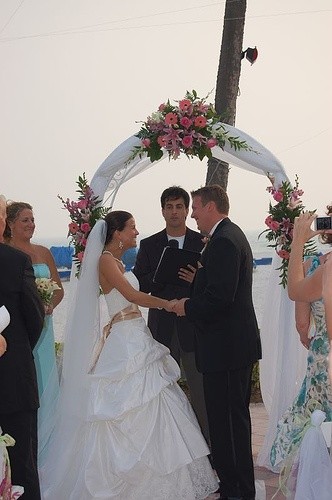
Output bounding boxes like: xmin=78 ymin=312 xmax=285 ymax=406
xmin=1 ymin=192 xmax=64 ymax=500
xmin=93 ymin=211 xmax=182 ymax=500
xmin=134 ymin=186 xmax=221 ymax=471
xmin=172 ymin=185 xmax=263 ymax=499
xmin=287 ymin=205 xmax=332 ymax=500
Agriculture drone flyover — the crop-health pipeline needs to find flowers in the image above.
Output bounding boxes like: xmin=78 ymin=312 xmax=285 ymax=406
xmin=258 ymin=177 xmax=318 ymax=290
xmin=32 ymin=277 xmax=61 ymax=309
xmin=200 ymin=236 xmax=209 ymax=244
xmin=58 ymin=175 xmax=98 ymax=263
xmin=129 ymin=88 xmax=226 ymax=161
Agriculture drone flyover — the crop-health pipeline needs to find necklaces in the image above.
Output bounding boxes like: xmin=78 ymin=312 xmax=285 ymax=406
xmin=102 ymin=250 xmax=127 ymax=269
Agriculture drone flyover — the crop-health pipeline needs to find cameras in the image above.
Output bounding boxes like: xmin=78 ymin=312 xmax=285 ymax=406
xmin=314 ymin=217 xmax=332 ymax=230
